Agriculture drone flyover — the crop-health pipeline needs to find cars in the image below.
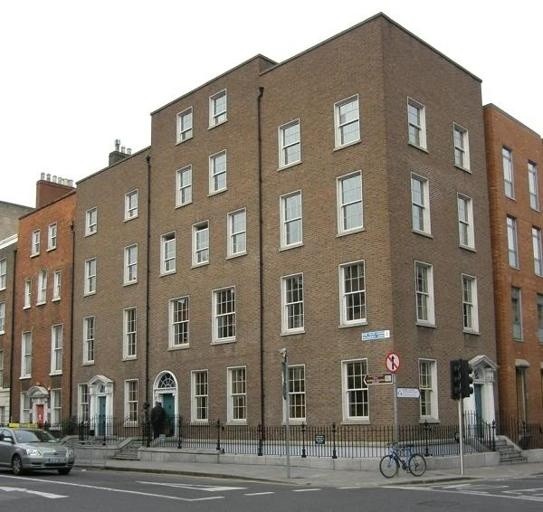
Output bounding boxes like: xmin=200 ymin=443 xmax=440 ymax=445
xmin=0 ymin=426 xmax=76 ymax=475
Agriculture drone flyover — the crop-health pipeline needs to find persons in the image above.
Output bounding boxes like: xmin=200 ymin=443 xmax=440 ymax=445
xmin=151 ymin=401 xmax=165 ymax=436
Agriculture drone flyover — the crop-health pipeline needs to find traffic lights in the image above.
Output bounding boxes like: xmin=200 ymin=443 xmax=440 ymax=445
xmin=450 ymin=360 xmax=462 ymax=399
xmin=464 ymin=360 xmax=473 ymax=395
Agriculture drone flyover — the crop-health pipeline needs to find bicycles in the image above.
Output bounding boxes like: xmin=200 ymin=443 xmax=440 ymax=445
xmin=378 ymin=441 xmax=427 ymax=477
